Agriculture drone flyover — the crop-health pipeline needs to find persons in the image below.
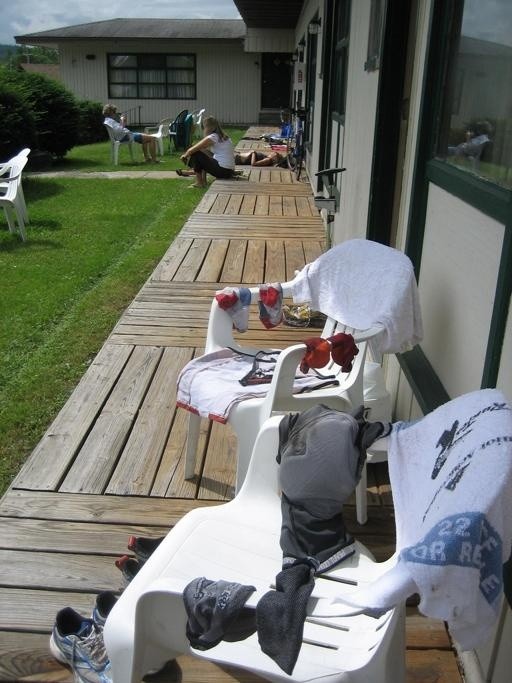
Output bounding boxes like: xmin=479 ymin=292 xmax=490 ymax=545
xmin=175 ymin=149 xmax=283 ymax=177
xmin=101 ymin=104 xmax=161 ymax=164
xmin=180 ymin=115 xmax=236 ymax=189
xmin=448 ymin=120 xmax=496 ymax=164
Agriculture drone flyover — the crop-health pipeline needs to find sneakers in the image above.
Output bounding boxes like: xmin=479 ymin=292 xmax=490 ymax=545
xmin=114 ymin=536 xmax=166 ymax=592
xmin=48 ymin=590 xmax=167 ymax=683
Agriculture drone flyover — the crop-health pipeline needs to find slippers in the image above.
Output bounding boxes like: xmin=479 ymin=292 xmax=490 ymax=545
xmin=175 ymin=167 xmax=189 ymax=178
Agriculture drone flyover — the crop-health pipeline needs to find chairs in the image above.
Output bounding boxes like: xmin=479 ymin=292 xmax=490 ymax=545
xmin=0 ymin=147 xmax=32 ymax=244
xmin=184 ymin=240 xmax=414 ymax=524
xmin=145 ymin=107 xmax=206 ymax=157
xmin=103 ymin=388 xmax=511 ymax=683
xmin=103 ymin=122 xmax=138 ymax=165
